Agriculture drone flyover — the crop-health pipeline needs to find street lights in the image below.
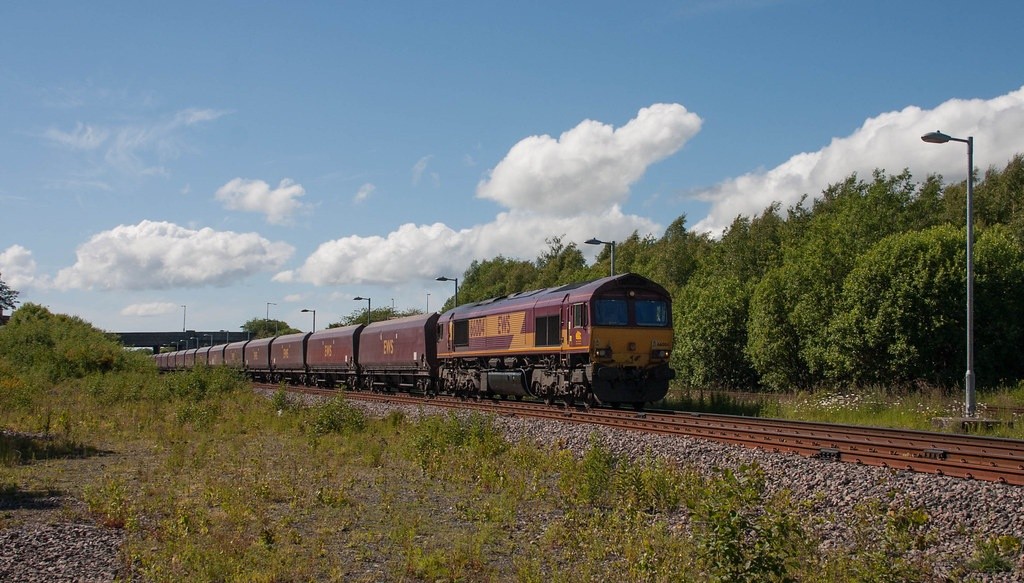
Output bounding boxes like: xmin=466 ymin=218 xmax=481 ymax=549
xmin=266 ymin=320 xmax=278 ymax=337
xmin=920 ymin=130 xmax=975 ymax=417
xmin=162 ymin=344 xmax=169 ymax=352
xmin=301 ymin=309 xmax=315 ymax=333
xmin=204 ymin=334 xmax=213 ymax=346
xmin=266 ymin=302 xmax=277 ymax=337
xmin=584 ymin=238 xmax=614 ymax=278
xmin=240 ymin=326 xmax=250 ymax=341
xmin=219 ymin=330 xmax=229 ymax=343
xmin=170 ymin=341 xmax=179 ymax=351
xmin=435 ymin=275 xmax=458 ymax=307
xmin=180 ymin=305 xmax=186 ymax=332
xmin=353 ymin=297 xmax=370 ymax=324
xmin=179 ymin=339 xmax=189 ymax=350
xmin=190 ymin=337 xmax=198 ymax=349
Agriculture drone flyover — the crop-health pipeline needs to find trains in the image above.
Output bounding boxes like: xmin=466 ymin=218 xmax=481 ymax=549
xmin=150 ymin=272 xmax=676 ymax=413
